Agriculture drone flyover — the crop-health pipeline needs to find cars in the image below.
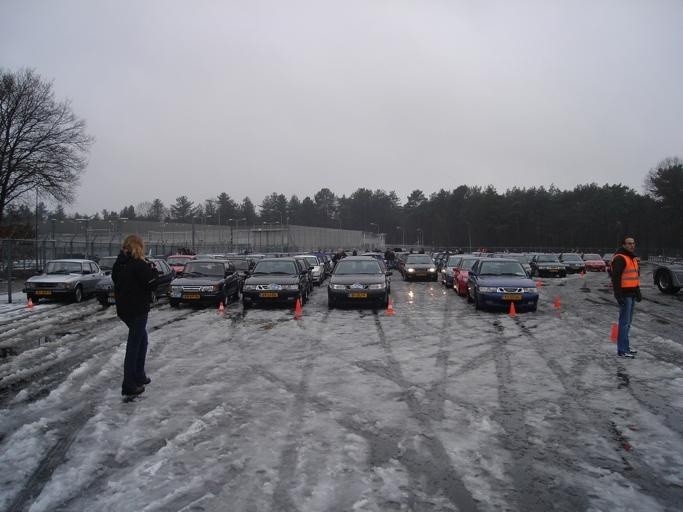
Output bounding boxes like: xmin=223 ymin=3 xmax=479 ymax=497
xmin=325 ymin=255 xmax=393 ymax=310
xmin=467 ymin=258 xmax=540 ymax=312
xmin=242 ymin=257 xmax=309 ymax=309
xmin=529 ymin=253 xmax=566 ymax=278
xmin=558 ymin=253 xmax=587 ymax=274
xmin=580 ymin=254 xmax=607 ymax=272
xmin=440 ymin=255 xmax=473 ymax=288
xmin=652 ymin=258 xmax=683 ymax=293
xmin=167 ymin=258 xmax=241 ymax=308
xmin=22 ymin=258 xmax=106 ymax=305
xmin=165 ymin=252 xmax=532 ymax=294
xmin=452 ymin=256 xmax=480 ymax=298
xmin=95 ymin=258 xmax=174 ymax=307
xmin=98 ymin=255 xmax=119 ymax=271
xmin=602 ymin=253 xmax=615 ymax=267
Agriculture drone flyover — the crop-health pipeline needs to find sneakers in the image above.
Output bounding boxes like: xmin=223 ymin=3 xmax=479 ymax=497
xmin=617 ymin=349 xmax=637 ymax=359
xmin=122 ymin=377 xmax=151 ymax=394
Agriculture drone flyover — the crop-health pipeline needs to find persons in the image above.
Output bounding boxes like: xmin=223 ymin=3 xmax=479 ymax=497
xmin=177 ymin=247 xmax=196 ymax=255
xmin=384 ymin=247 xmax=394 ymax=261
xmin=611 ymin=235 xmax=642 ymax=359
xmin=111 ymin=234 xmax=159 ymax=396
xmin=332 ymin=247 xmax=357 ymax=270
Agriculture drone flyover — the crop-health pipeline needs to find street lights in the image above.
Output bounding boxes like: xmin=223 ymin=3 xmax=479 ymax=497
xmin=229 ymin=217 xmax=246 ymax=248
xmin=371 ymin=222 xmax=380 ymax=233
xmin=263 ymin=221 xmax=280 ymax=230
xmin=417 ymin=228 xmax=425 ymax=245
xmin=397 ymin=226 xmax=405 ymax=245
xmin=192 ymin=214 xmax=213 ymax=245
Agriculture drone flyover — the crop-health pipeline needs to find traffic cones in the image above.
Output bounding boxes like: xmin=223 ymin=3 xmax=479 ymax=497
xmin=385 ymin=298 xmax=396 ymax=315
xmin=610 ymin=321 xmax=618 ymax=341
xmin=295 ymin=299 xmax=302 ymax=313
xmin=508 ymin=301 xmax=517 ymax=316
